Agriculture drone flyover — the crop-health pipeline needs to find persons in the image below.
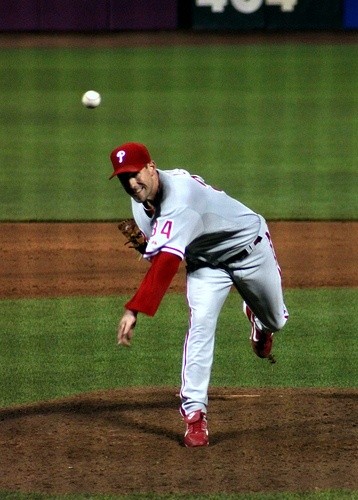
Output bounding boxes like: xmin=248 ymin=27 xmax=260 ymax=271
xmin=109 ymin=142 xmax=289 ymax=449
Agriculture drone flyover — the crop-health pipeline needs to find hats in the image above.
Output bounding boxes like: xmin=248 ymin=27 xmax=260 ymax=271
xmin=109 ymin=142 xmax=150 ymax=179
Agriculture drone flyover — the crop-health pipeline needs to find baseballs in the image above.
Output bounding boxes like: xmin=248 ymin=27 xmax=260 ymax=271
xmin=82 ymin=91 xmax=101 ymax=109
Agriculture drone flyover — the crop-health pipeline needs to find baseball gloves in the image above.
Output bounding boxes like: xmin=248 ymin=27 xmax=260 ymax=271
xmin=116 ymin=217 xmax=146 ymax=257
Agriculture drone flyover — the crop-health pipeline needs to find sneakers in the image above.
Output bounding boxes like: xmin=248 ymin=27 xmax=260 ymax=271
xmin=183 ymin=409 xmax=210 ymax=447
xmin=242 ymin=300 xmax=273 ymax=358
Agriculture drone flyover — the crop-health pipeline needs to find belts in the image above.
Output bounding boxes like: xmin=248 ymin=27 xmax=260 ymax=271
xmin=224 ymin=235 xmax=262 ymax=264
